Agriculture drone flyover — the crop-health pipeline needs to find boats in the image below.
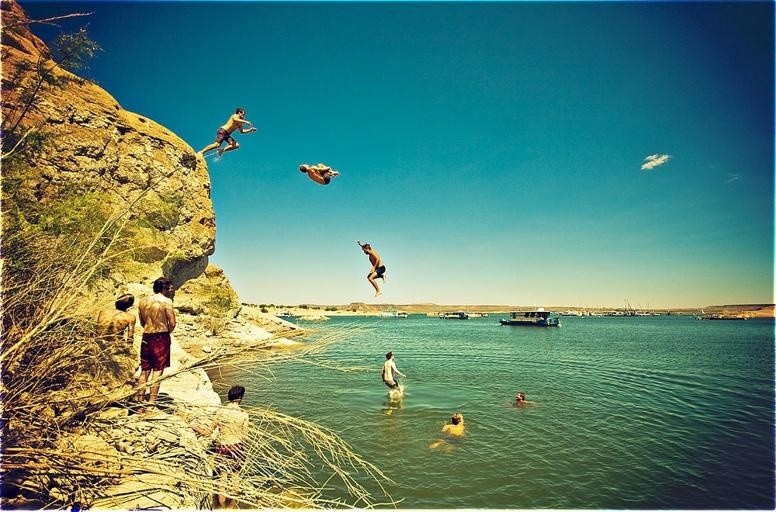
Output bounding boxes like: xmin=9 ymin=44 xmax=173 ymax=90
xmin=265 ymin=338 xmax=305 ymax=348
xmin=296 ymin=315 xmax=330 ymax=321
xmin=427 ymin=311 xmax=488 ymax=318
xmin=276 ymin=312 xmax=294 ymax=316
xmin=379 ymin=312 xmax=408 ymax=318
xmin=500 ymin=311 xmax=559 ymax=327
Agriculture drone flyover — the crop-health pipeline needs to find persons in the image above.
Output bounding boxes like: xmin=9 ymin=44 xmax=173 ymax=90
xmin=426 ymin=413 xmax=465 ymax=453
xmin=136 ymin=276 xmax=176 ymax=414
xmin=299 ymin=162 xmax=341 ymax=186
xmin=194 ymin=384 xmax=250 ymax=509
xmin=92 ymin=290 xmax=138 ymax=357
xmin=507 ymin=391 xmax=539 ymax=409
xmin=357 ymin=240 xmax=387 ymax=298
xmin=380 ymin=351 xmax=407 ymax=396
xmin=197 ymin=107 xmax=257 ymax=160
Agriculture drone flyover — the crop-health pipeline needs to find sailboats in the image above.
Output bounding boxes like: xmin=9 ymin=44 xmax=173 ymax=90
xmin=562 ymin=299 xmax=660 ymax=316
